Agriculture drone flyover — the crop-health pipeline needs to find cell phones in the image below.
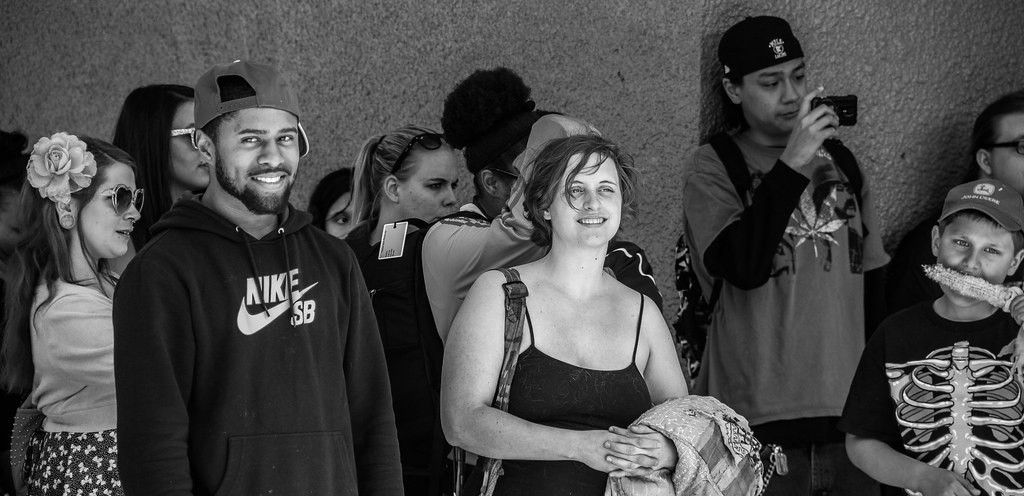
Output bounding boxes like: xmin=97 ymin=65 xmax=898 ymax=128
xmin=811 ymin=95 xmax=858 ymax=127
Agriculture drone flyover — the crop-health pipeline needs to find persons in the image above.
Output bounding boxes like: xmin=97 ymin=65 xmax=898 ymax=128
xmin=970 ymin=90 xmax=1024 ymax=197
xmin=422 ymin=68 xmax=602 ymax=464
xmin=441 ymin=136 xmax=691 ymax=496
xmin=112 ymin=60 xmax=404 ymax=496
xmin=307 ymin=167 xmax=354 ymax=240
xmin=0 ymin=132 xmax=141 ymax=496
xmin=345 ymin=123 xmax=461 ymax=496
xmin=0 ymin=127 xmax=33 ymax=313
xmin=839 ymin=178 xmax=1024 ymax=496
xmin=683 ymin=16 xmax=891 ymax=496
xmin=112 ymin=84 xmax=210 ymax=253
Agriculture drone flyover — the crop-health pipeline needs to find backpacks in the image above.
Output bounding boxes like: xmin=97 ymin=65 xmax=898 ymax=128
xmin=671 ymin=131 xmax=869 ymax=380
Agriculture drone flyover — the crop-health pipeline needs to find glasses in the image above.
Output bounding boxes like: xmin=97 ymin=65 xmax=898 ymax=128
xmin=169 ymin=128 xmax=198 ymax=149
xmin=988 ymin=140 xmax=1024 ymax=154
xmin=101 ymin=183 xmax=144 ymax=216
xmin=390 ymin=133 xmax=444 ymax=176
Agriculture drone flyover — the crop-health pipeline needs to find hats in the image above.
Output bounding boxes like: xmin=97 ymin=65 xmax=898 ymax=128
xmin=193 ymin=60 xmax=309 ymax=158
xmin=937 ymin=178 xmax=1024 ymax=232
